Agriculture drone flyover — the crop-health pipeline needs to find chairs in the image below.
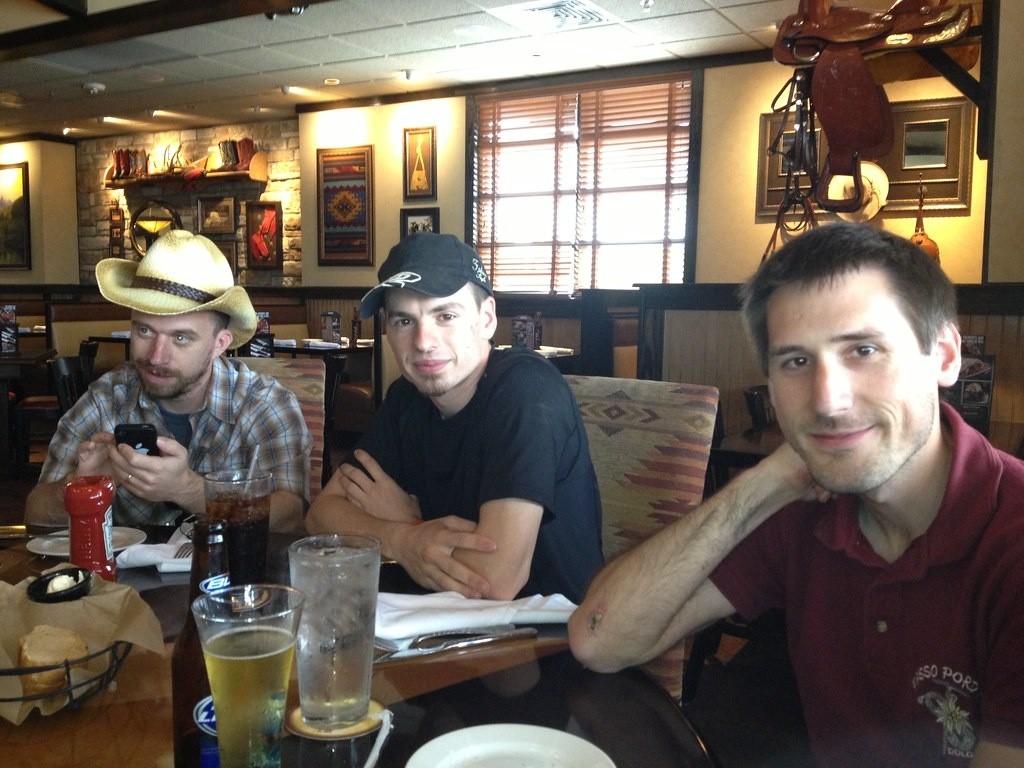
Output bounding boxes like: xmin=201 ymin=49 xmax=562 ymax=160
xmin=15 ymin=338 xmax=100 ymax=482
xmin=233 ymin=355 xmax=332 ymax=506
xmin=557 ymin=373 xmax=716 ymax=709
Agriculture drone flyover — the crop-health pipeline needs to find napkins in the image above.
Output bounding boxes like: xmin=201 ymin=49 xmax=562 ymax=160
xmin=373 ymin=590 xmax=577 ymax=651
xmin=115 ymin=522 xmax=193 ymax=571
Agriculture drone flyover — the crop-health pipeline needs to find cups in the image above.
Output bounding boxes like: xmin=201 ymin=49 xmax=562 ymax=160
xmin=287 ymin=532 xmax=383 ymax=725
xmin=190 ymin=583 xmax=306 ymax=768
xmin=352 ymin=320 xmax=361 ymax=342
xmin=202 ymin=468 xmax=273 ymax=586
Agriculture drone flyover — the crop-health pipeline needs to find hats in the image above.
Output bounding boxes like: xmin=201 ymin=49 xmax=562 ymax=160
xmin=357 ymin=230 xmax=497 ymax=321
xmin=93 ymin=228 xmax=259 ymax=351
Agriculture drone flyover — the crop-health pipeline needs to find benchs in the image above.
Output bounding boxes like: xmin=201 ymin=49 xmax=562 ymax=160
xmin=339 ymin=307 xmax=403 ymax=435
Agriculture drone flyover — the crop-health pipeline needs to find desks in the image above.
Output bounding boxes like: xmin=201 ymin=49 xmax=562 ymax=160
xmin=89 ymin=333 xmax=376 ymax=394
xmin=273 ymin=338 xmax=373 ymax=430
xmin=0 ymin=346 xmax=57 ymax=457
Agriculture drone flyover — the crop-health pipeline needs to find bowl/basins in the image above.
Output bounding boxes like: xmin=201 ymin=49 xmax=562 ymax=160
xmin=26 ymin=567 xmax=93 ymax=603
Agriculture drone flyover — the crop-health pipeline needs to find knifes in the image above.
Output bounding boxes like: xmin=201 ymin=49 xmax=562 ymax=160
xmin=389 ymin=627 xmax=538 ymax=658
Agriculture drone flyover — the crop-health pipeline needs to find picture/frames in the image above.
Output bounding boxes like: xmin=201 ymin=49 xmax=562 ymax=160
xmin=210 ymin=237 xmax=238 ymax=281
xmin=403 ymin=125 xmax=438 ymax=201
xmin=0 ymin=161 xmax=30 ymax=269
xmin=316 ymin=144 xmax=376 ymax=267
xmin=109 ymin=208 xmax=125 ymax=258
xmin=399 ymin=206 xmax=440 ymax=242
xmin=245 ymin=200 xmax=284 ymax=270
xmin=197 ymin=196 xmax=236 ymax=233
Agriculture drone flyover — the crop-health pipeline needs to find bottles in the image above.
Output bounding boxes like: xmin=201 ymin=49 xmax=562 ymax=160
xmin=171 ymin=518 xmax=232 ymax=768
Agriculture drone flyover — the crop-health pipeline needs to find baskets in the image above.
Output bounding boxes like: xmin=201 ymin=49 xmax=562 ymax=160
xmin=0 ymin=588 xmax=138 ymax=720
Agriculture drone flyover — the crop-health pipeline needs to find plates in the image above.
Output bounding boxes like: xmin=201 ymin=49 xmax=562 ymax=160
xmin=404 ymin=723 xmax=618 ymax=768
xmin=26 ymin=526 xmax=148 ymax=557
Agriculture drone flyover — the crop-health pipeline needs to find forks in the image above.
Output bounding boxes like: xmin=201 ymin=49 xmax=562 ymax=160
xmin=373 ymin=624 xmax=516 ymax=653
xmin=174 ymin=543 xmax=194 ymax=558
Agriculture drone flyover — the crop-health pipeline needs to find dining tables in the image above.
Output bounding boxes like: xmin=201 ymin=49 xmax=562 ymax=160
xmin=0 ymin=517 xmax=716 ymax=768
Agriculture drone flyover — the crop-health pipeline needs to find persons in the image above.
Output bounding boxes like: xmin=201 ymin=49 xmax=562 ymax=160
xmin=22 ymin=229 xmax=312 ymax=536
xmin=567 ymin=225 xmax=1023 ymax=768
xmin=302 ymin=232 xmax=602 ymax=699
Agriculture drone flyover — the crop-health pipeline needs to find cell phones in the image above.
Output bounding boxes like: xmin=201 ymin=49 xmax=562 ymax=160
xmin=115 ymin=423 xmax=160 ymax=457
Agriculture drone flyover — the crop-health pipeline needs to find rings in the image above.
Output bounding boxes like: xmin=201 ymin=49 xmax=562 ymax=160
xmin=125 ymin=474 xmax=132 ymax=482
